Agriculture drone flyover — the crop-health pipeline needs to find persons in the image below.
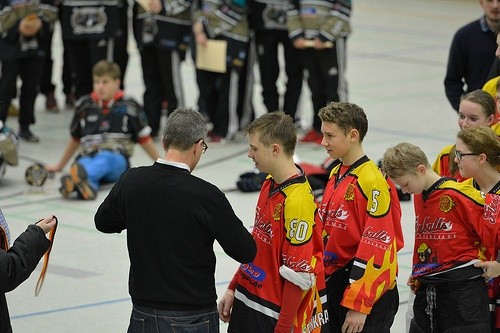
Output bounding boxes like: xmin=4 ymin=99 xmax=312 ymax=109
xmin=0 ymin=209 xmax=57 ymax=333
xmin=318 ymin=101 xmax=405 ymax=333
xmin=1 ymin=0 xmax=353 ymax=146
xmin=218 ymin=111 xmax=330 ymax=333
xmin=481 ymin=30 xmax=500 ymax=102
xmin=430 ymin=89 xmax=497 ymax=184
xmin=94 ymin=106 xmax=258 ymax=333
xmin=442 ymin=0 xmax=500 ymax=115
xmin=381 ymin=142 xmax=500 ymax=333
xmin=490 ymin=78 xmax=500 ymax=142
xmin=42 ymin=58 xmax=163 ymax=200
xmin=453 ymin=125 xmax=500 ymax=333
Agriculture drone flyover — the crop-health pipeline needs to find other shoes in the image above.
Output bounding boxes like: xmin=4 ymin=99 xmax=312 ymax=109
xmin=46 ymin=103 xmax=58 ymax=113
xmin=66 ymin=96 xmax=77 ymax=106
xmin=206 ymin=135 xmax=226 ymax=145
xmin=59 ymin=175 xmax=73 ymax=197
xmin=19 ymin=131 xmax=37 ymax=141
xmin=70 ymin=164 xmax=96 ymax=200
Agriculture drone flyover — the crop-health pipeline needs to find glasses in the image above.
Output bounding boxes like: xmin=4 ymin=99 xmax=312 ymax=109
xmin=196 ymin=139 xmax=208 ymax=153
xmin=454 ymin=150 xmax=488 ymax=161
